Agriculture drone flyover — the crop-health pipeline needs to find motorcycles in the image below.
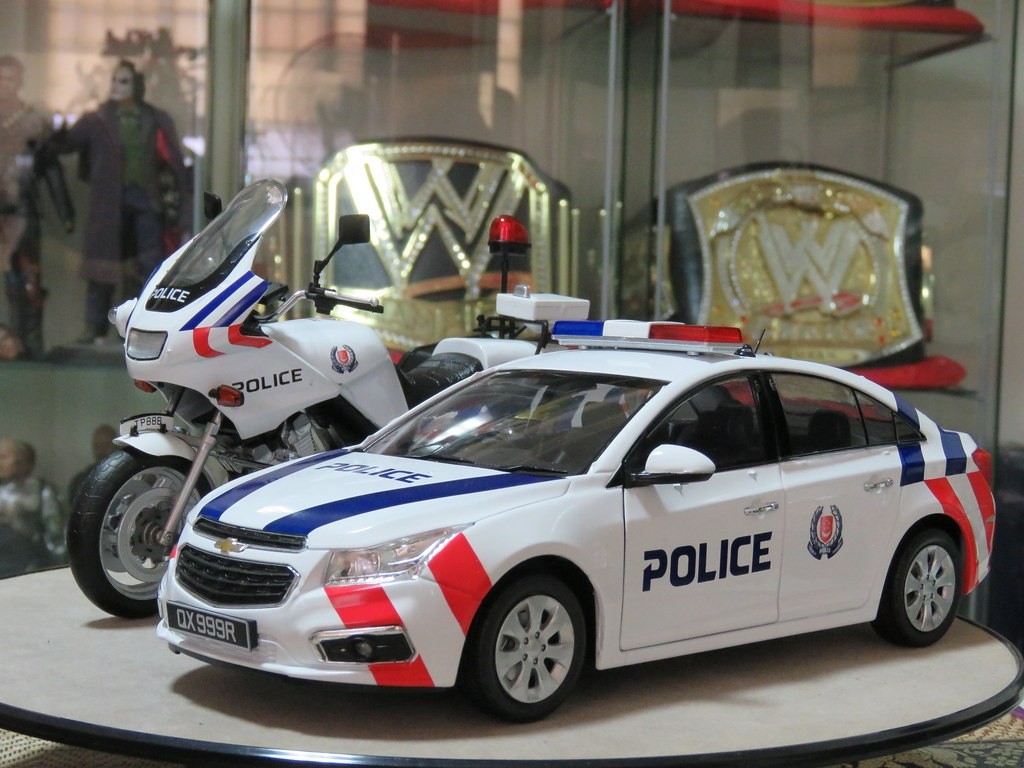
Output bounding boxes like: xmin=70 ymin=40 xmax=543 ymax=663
xmin=66 ymin=179 xmax=592 ymax=620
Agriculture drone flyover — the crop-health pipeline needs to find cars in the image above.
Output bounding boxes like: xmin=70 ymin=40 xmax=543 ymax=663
xmin=157 ymin=317 xmax=997 ymax=723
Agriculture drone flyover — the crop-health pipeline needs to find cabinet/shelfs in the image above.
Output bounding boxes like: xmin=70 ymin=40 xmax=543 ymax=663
xmin=1 ymin=4 xmax=1024 ymax=631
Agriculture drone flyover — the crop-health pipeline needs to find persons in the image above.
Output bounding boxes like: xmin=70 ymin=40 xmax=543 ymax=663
xmin=1 ymin=54 xmax=192 ymax=578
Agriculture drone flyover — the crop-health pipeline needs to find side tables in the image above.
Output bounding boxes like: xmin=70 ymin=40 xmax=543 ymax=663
xmin=1 ymin=566 xmax=1023 ymax=768
